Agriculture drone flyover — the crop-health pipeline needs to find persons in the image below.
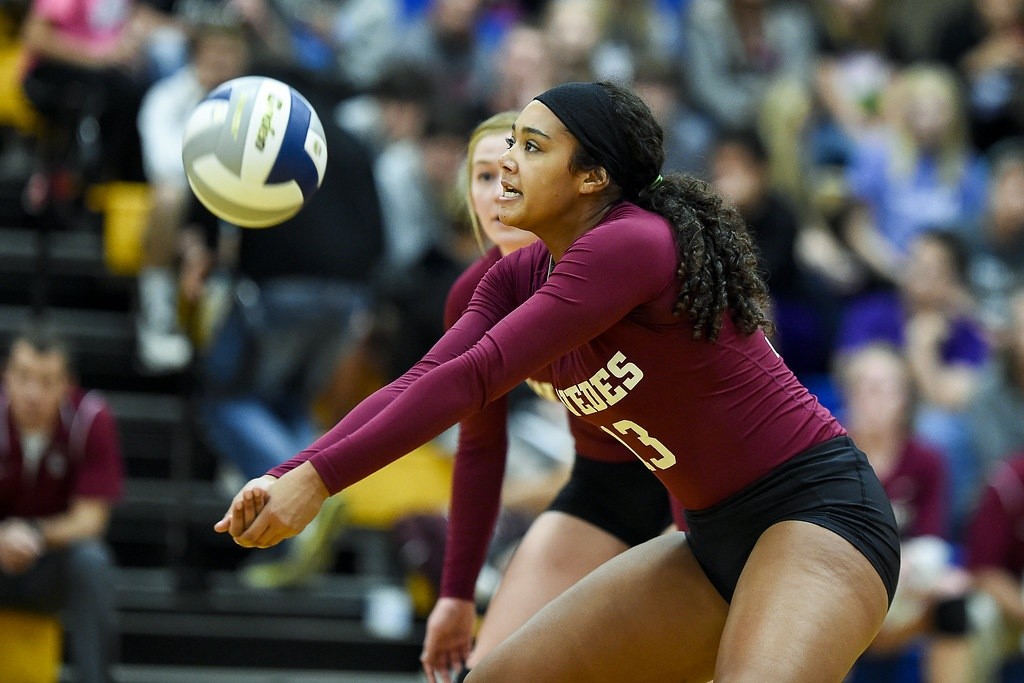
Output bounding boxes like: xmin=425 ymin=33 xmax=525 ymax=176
xmin=213 ymin=79 xmax=902 ymax=681
xmin=418 ymin=112 xmax=674 ymax=681
xmin=0 ymin=0 xmax=1024 ymax=683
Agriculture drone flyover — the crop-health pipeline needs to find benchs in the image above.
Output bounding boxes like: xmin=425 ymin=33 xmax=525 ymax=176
xmin=0 ymin=155 xmax=430 ymax=683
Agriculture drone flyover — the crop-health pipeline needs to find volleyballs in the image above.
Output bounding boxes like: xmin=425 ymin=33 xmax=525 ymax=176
xmin=182 ymin=76 xmax=327 ymax=229
